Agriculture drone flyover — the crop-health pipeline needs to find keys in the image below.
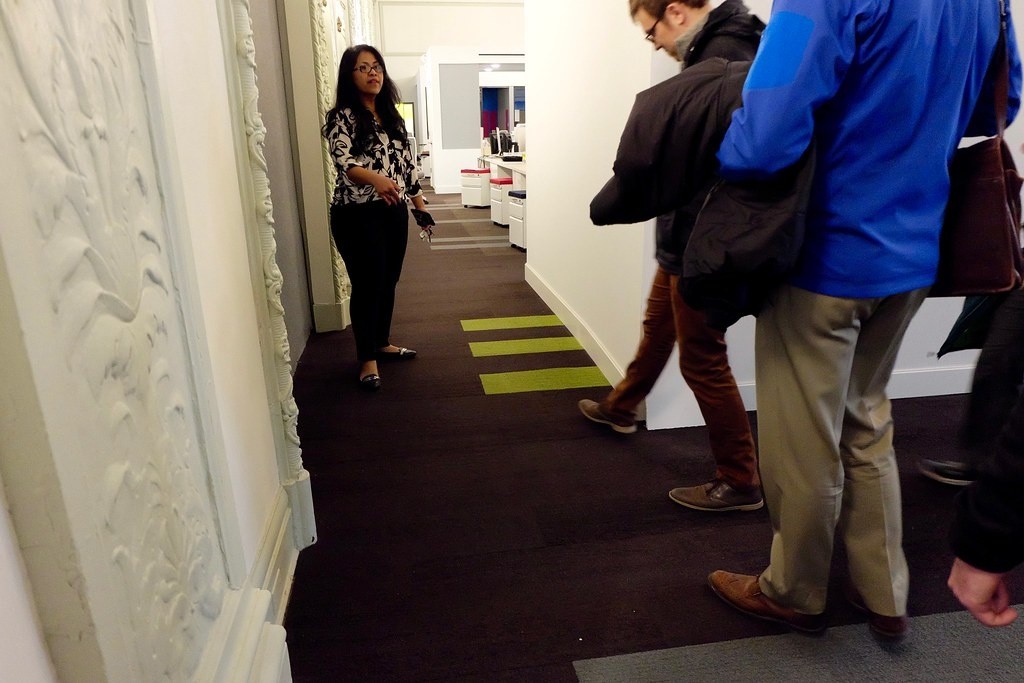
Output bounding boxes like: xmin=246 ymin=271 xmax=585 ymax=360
xmin=427 ymin=228 xmax=433 ymax=243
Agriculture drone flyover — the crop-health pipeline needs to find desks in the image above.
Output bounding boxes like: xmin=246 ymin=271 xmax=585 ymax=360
xmin=477 ymin=156 xmax=527 ymax=191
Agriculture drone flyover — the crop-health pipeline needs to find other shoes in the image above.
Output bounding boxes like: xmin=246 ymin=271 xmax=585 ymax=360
xmin=381 ymin=347 xmax=416 ymax=358
xmin=359 ymin=373 xmax=382 ymax=392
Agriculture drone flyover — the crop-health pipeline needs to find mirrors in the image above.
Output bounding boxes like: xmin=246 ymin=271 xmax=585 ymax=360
xmin=480 ymin=54 xmax=525 ymax=165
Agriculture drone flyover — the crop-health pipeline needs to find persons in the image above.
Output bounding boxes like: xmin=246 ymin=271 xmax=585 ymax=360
xmin=708 ymin=0 xmax=1024 ymax=646
xmin=576 ymin=0 xmax=765 ymax=512
xmin=322 ymin=45 xmax=433 ymax=392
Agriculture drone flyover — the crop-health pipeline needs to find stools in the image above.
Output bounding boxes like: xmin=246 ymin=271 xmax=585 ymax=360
xmin=461 ymin=168 xmax=490 ymax=208
xmin=507 ymin=190 xmax=527 ymax=251
xmin=490 ymin=177 xmax=512 ymax=228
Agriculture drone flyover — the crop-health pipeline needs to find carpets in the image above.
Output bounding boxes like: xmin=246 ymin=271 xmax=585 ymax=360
xmin=573 ymin=603 xmax=1023 ymax=683
xmin=469 ymin=336 xmax=584 ymax=357
xmin=480 ymin=366 xmax=611 ymax=394
xmin=459 ymin=314 xmax=565 ymax=332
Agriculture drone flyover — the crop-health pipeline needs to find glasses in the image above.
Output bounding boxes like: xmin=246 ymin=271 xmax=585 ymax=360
xmin=353 ymin=64 xmax=384 ymax=73
xmin=644 ymin=15 xmax=663 ymax=43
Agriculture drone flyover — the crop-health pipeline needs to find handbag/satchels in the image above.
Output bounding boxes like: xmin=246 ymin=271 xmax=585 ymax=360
xmin=927 ymin=136 xmax=1024 ymax=296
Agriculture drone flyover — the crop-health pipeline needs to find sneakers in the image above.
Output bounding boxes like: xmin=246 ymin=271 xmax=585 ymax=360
xmin=669 ymin=477 xmax=764 ymax=511
xmin=918 ymin=456 xmax=982 ymax=485
xmin=708 ymin=570 xmax=827 ymax=637
xmin=840 ymin=572 xmax=910 ymax=643
xmin=578 ymin=398 xmax=636 ymax=433
